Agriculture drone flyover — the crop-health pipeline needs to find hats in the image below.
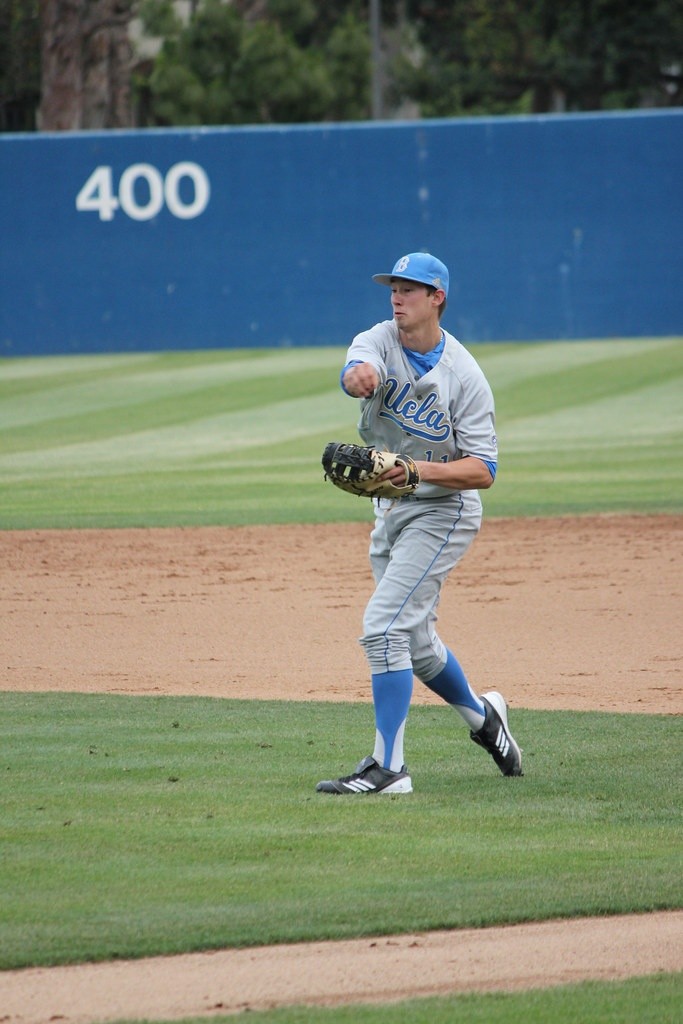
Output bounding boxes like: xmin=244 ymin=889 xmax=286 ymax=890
xmin=372 ymin=252 xmax=449 ymax=299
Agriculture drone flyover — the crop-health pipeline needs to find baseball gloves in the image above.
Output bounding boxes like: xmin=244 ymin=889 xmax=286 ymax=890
xmin=320 ymin=440 xmax=421 ymax=512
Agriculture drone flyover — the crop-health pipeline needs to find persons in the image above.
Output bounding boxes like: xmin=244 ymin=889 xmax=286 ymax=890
xmin=317 ymin=253 xmax=523 ymax=797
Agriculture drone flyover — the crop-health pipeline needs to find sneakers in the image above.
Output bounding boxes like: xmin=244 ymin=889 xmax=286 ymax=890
xmin=316 ymin=756 xmax=414 ymax=794
xmin=470 ymin=691 xmax=522 ymax=777
